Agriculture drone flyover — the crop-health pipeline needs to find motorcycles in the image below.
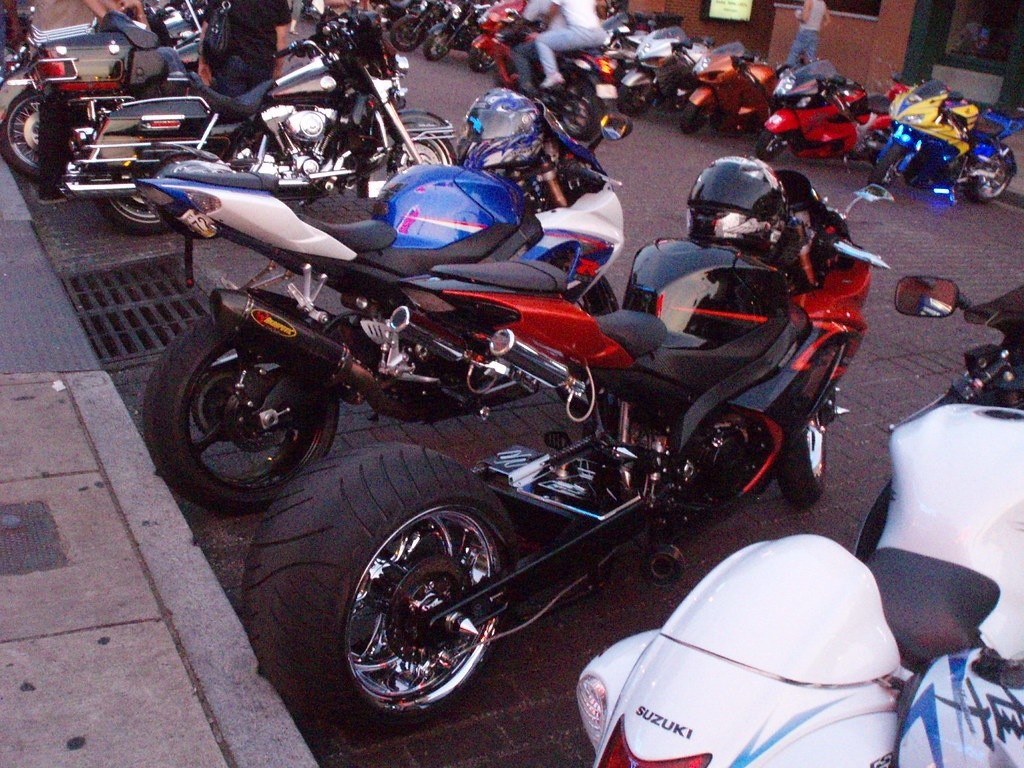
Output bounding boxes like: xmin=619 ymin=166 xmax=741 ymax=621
xmin=679 ymin=35 xmax=797 ymax=138
xmin=574 ymin=273 xmax=1024 ymax=768
xmin=614 ymin=13 xmax=717 ymax=118
xmin=238 ymin=151 xmax=895 ymax=741
xmin=866 ymin=69 xmax=1024 ymax=208
xmin=753 ymin=49 xmax=913 ymax=164
xmin=0 ymin=1 xmax=685 ymax=240
xmin=120 ymin=75 xmax=635 ymax=514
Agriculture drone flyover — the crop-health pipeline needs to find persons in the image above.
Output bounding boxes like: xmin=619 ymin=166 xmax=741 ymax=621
xmin=36 ymin=0 xmax=151 ymax=31
xmin=511 ymin=0 xmax=606 ymax=94
xmin=786 ymin=0 xmax=830 ymax=65
xmin=199 ymin=0 xmax=291 ymax=99
xmin=287 ymin=0 xmax=303 ymax=36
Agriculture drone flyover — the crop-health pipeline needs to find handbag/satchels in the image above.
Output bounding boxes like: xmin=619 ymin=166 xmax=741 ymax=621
xmin=202 ymin=0 xmax=233 ymax=63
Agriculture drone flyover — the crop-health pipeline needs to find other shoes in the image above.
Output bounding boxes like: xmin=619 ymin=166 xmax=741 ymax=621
xmin=38 ymin=187 xmax=68 ymax=204
xmin=540 ymin=72 xmax=563 ymax=89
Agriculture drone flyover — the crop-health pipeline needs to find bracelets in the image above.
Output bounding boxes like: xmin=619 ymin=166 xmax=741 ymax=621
xmin=138 ymin=14 xmax=146 ymax=18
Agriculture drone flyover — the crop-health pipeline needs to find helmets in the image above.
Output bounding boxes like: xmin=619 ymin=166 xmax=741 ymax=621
xmin=463 ymin=88 xmax=545 ymax=171
xmin=688 ymin=155 xmax=788 ymax=248
xmin=891 ymin=647 xmax=1024 ymax=768
xmin=544 ymin=77 xmax=606 ymax=141
